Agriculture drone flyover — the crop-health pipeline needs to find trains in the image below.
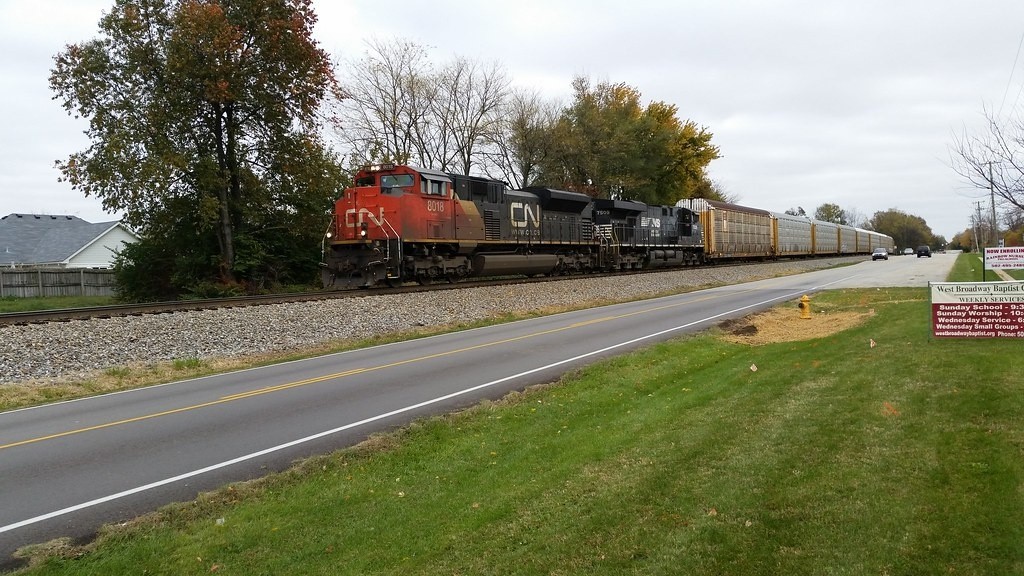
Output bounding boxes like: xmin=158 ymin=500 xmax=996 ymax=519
xmin=317 ymin=160 xmax=895 ymax=290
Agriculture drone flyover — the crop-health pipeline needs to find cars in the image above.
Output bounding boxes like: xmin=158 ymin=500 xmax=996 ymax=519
xmin=916 ymin=246 xmax=931 ymax=258
xmin=872 ymin=248 xmax=888 ymax=261
xmin=904 ymin=247 xmax=914 ymax=255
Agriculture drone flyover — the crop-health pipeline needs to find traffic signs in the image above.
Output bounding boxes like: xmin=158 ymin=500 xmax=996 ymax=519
xmin=984 ymin=247 xmax=1024 ymax=270
